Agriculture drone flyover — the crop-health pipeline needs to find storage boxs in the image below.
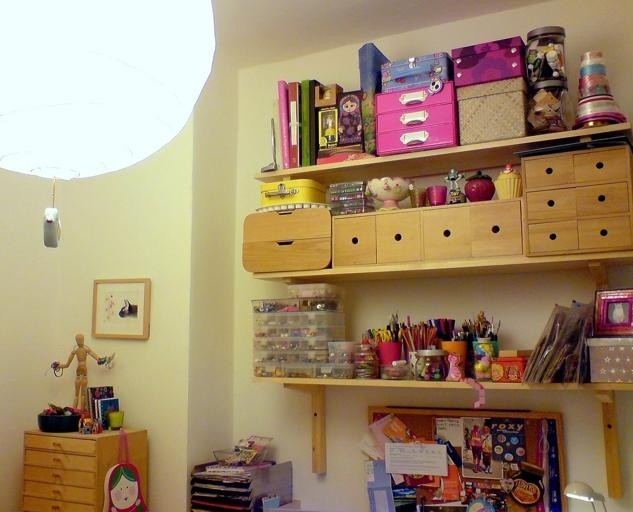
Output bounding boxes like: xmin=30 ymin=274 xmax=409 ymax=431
xmin=449 ymin=34 xmax=526 ymax=87
xmin=238 ymin=204 xmax=334 ymax=272
xmin=516 ymin=142 xmax=632 ymax=260
xmin=328 ymin=193 xmax=529 ymax=270
xmin=486 ymin=355 xmax=534 ymax=384
xmin=378 ymin=51 xmax=452 ymax=93
xmin=258 ymin=175 xmax=329 ymax=209
xmin=452 ymin=78 xmax=532 ymax=146
xmin=585 ymin=332 xmax=632 ymax=387
xmin=250 ymin=292 xmax=348 ymax=380
xmin=370 ymin=81 xmax=461 ymax=159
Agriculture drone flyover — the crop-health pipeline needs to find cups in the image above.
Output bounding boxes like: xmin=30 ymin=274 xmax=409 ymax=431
xmin=408 ymin=185 xmax=448 ymax=208
xmin=107 ymin=412 xmax=124 ymax=431
xmin=377 ymin=342 xmax=400 ymax=367
xmin=472 ymin=341 xmax=499 ymax=380
xmin=441 ymin=340 xmax=467 ymax=378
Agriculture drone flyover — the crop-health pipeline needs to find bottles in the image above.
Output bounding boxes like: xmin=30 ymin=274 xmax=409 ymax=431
xmin=524 ymin=26 xmax=572 ymax=133
xmin=414 ymin=349 xmax=449 ymax=381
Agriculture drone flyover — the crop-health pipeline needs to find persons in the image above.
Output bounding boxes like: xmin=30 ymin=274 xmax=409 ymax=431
xmin=462 ymin=426 xmax=472 ymax=464
xmin=470 ymin=424 xmax=482 ymax=475
xmin=51 ymin=333 xmax=112 ymax=413
xmin=481 ymin=426 xmax=492 ymax=474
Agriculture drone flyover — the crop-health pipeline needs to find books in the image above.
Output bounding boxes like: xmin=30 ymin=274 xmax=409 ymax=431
xmin=300 ymin=79 xmax=310 ymax=169
xmin=310 ymin=78 xmax=323 ymax=168
xmin=275 ymin=77 xmax=288 ymax=167
xmin=356 ymin=41 xmax=388 ymax=153
xmin=289 ymin=80 xmax=300 ymax=169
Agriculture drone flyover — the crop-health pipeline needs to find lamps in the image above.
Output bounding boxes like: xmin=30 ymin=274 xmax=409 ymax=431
xmin=0 ymin=0 xmax=216 ymax=185
xmin=561 ymin=472 xmax=614 ymax=511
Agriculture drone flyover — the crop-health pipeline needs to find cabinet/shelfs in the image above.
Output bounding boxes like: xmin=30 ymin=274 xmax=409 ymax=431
xmin=246 ymin=121 xmax=632 ymax=285
xmin=19 ymin=423 xmax=150 ymax=510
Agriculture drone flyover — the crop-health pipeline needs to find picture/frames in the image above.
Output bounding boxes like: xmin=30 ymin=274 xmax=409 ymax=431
xmin=591 ymin=285 xmax=632 ymax=339
xmin=89 ymin=277 xmax=151 ymax=341
xmin=362 ymin=400 xmax=571 ymax=512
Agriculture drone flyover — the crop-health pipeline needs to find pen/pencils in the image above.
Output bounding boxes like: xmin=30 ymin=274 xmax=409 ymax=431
xmin=427 ymin=319 xmax=455 ymax=341
xmin=453 ymin=330 xmax=465 ymax=341
xmin=465 ymin=312 xmax=501 ymax=342
xmin=400 ymin=316 xmax=438 ymax=352
